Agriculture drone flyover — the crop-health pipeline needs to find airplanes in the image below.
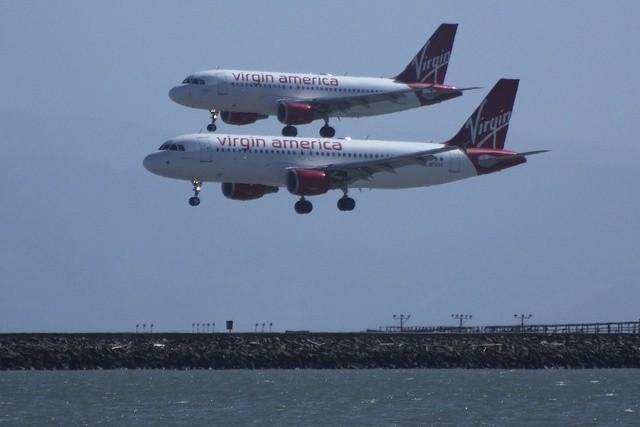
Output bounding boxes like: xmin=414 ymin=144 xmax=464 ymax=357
xmin=168 ymin=23 xmax=485 ymax=138
xmin=142 ymin=78 xmax=554 ymax=214
